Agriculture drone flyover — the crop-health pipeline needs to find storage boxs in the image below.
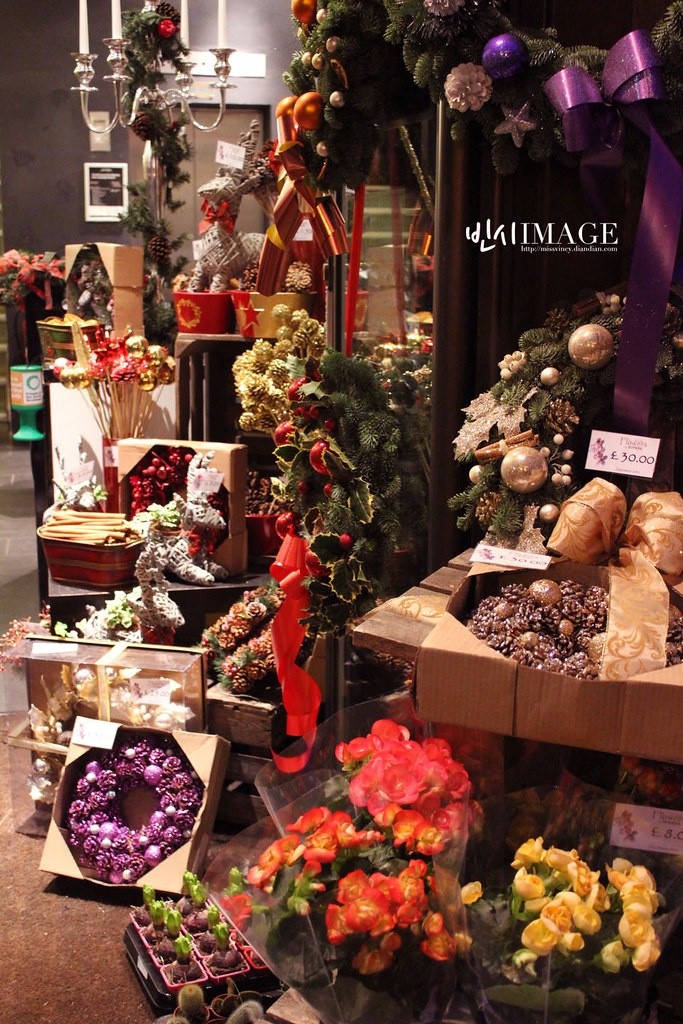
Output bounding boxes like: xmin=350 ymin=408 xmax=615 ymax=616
xmin=64 ymin=243 xmax=146 ymax=346
xmin=367 ymin=247 xmax=405 ymax=335
xmin=37 ymin=717 xmax=234 ymax=899
xmin=117 ymin=439 xmax=248 ymax=573
xmin=0 ymin=632 xmax=208 ymax=838
xmin=412 ymin=546 xmax=683 ymax=762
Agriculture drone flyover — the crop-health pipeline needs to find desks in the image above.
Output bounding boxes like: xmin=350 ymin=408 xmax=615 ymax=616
xmin=48 ymin=563 xmax=276 ymax=647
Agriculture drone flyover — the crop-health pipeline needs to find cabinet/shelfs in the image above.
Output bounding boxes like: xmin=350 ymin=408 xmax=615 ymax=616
xmin=175 ymin=331 xmax=243 ymax=441
xmin=49 ymin=381 xmax=178 ymax=506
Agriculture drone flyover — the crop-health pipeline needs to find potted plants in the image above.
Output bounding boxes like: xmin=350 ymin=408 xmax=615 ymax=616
xmin=245 ymin=472 xmax=285 ymax=564
xmin=232 ymin=255 xmax=316 ymax=340
xmin=130 ymin=867 xmax=274 ymax=1024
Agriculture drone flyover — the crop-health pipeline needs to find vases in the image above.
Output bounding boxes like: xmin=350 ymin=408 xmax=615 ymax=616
xmin=169 ymin=289 xmax=231 ymax=334
xmin=102 ymin=435 xmax=117 ymax=513
xmin=235 ymin=431 xmax=285 ymax=472
xmin=353 ymin=291 xmax=369 ymax=333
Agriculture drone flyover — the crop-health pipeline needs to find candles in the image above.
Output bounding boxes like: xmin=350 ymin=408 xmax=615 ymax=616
xmin=218 ymin=0 xmax=227 ymax=48
xmin=109 ymin=0 xmax=126 ymax=39
xmin=179 ymin=0 xmax=190 ymax=50
xmin=75 ymin=0 xmax=90 ymax=55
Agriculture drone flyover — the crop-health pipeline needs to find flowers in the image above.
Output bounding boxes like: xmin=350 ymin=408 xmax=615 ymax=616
xmin=68 ymin=741 xmax=202 ymax=885
xmin=422 ymin=0 xmax=465 ymax=19
xmin=467 ymin=576 xmax=683 ymax=681
xmin=250 ymin=728 xmax=683 ymax=1024
xmin=229 ymin=304 xmax=327 ymax=432
xmin=27 ymin=665 xmax=194 ymax=823
xmin=199 ymin=583 xmax=311 ymax=695
xmin=446 ymin=283 xmax=683 ymax=553
xmin=51 ymin=325 xmax=175 ymax=440
xmin=442 ymin=62 xmax=493 ymax=116
